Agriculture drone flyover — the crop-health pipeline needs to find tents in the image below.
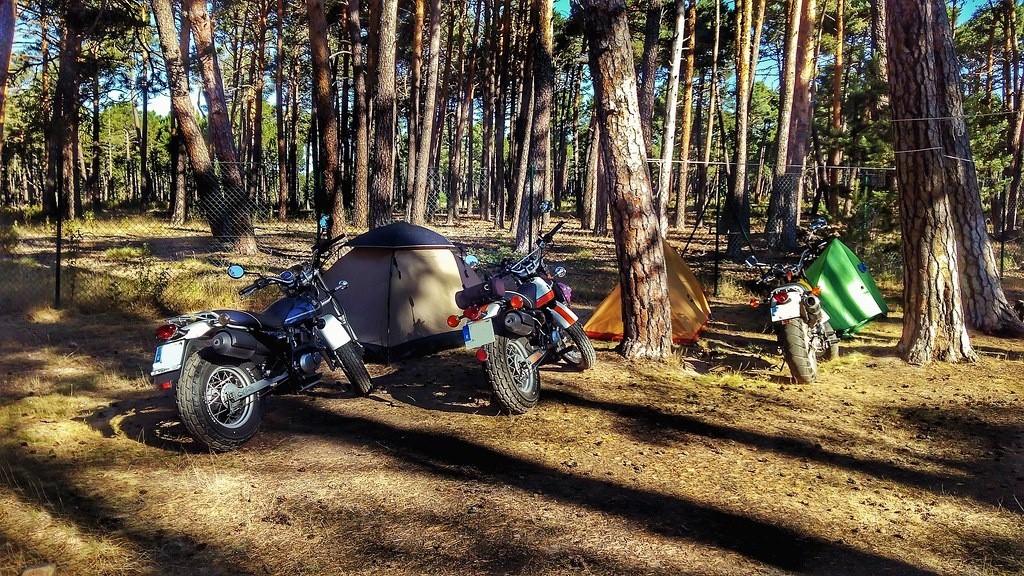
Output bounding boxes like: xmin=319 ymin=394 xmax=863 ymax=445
xmin=322 ymin=220 xmax=485 ymax=363
xmin=582 ymin=238 xmax=715 ymax=346
xmin=806 ymin=237 xmax=889 ymax=340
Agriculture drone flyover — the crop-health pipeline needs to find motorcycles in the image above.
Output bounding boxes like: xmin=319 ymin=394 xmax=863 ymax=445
xmin=151 ymin=213 xmax=377 ymax=455
xmin=446 ymin=200 xmax=595 ymax=417
xmin=745 ymin=218 xmax=842 ymax=384
